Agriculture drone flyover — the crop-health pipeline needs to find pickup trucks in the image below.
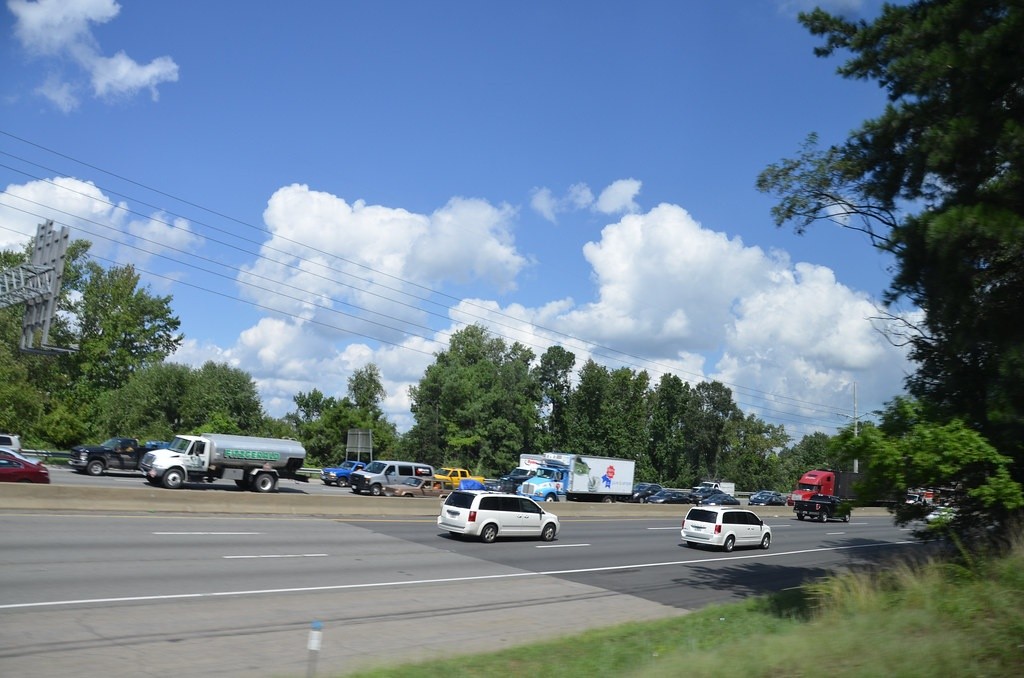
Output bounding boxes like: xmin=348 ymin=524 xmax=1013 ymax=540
xmin=70 ymin=437 xmax=162 ymax=476
xmin=793 ymin=494 xmax=850 ymax=522
xmin=434 ymin=467 xmax=484 ymax=487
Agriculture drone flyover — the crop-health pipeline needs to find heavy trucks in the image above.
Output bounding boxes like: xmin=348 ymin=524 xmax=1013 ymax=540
xmin=789 ymin=468 xmax=902 ymax=507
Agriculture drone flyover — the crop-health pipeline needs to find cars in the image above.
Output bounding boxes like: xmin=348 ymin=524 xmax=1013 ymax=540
xmin=1 ymin=433 xmax=21 ymax=454
xmin=0 ymin=448 xmax=51 ymax=485
xmin=907 ymin=491 xmax=947 ymax=524
xmin=630 ymin=480 xmax=786 ymax=506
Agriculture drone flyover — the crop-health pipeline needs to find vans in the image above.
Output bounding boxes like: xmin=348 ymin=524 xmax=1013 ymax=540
xmin=350 ymin=460 xmax=434 ymax=497
xmin=382 ymin=477 xmax=455 ymax=499
xmin=680 ymin=506 xmax=771 ymax=551
xmin=436 ymin=489 xmax=560 ymax=543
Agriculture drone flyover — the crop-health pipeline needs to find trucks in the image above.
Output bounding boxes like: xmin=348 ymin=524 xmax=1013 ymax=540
xmin=140 ymin=432 xmax=308 ymax=494
xmin=500 ymin=453 xmax=545 ymax=493
xmin=320 ymin=460 xmax=366 ymax=488
xmin=516 ymin=453 xmax=636 ymax=504
